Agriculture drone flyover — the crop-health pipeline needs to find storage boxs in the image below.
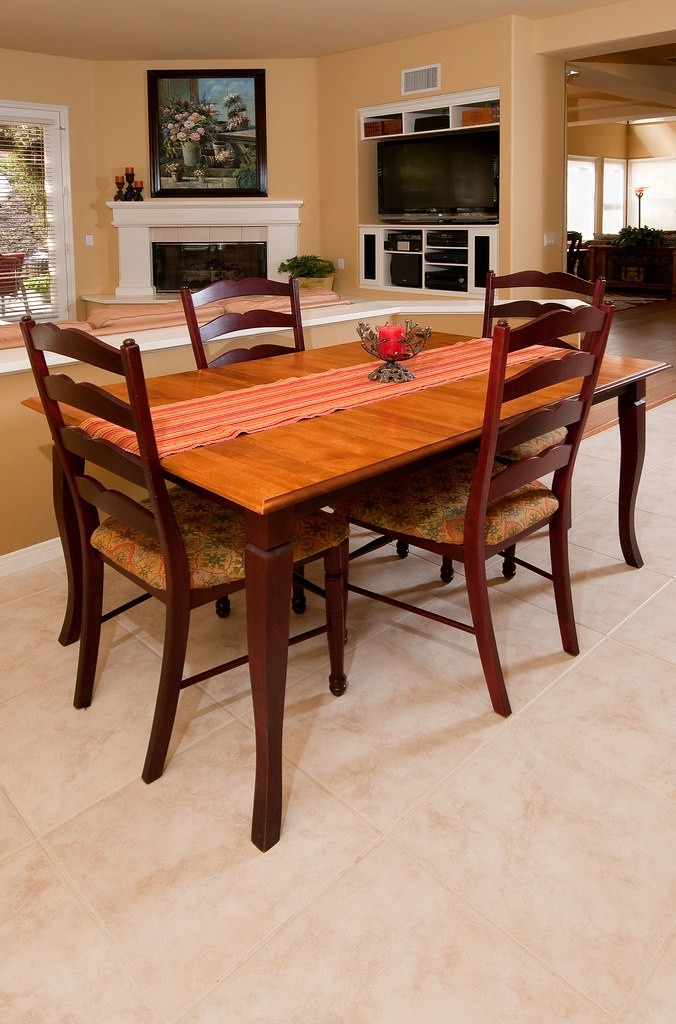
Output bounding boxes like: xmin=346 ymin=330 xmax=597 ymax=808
xmin=621 ymin=267 xmax=646 ymax=283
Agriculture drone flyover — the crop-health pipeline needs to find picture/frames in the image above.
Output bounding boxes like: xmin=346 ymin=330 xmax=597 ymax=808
xmin=147 ymin=69 xmax=268 ymax=198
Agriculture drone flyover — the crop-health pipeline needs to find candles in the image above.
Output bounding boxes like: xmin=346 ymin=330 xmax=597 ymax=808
xmin=125 ymin=166 xmax=134 ymax=174
xmin=134 ymin=180 xmax=143 ymax=188
xmin=115 ymin=176 xmax=124 ymax=182
xmin=379 ymin=326 xmax=406 ymax=360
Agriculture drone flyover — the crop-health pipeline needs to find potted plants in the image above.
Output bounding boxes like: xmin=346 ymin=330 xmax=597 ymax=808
xmin=278 ymin=255 xmax=337 ymax=291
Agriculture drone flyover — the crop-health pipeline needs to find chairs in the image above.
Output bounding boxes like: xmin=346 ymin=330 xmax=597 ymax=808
xmin=180 ymin=274 xmax=305 ymax=369
xmin=0 ymin=252 xmax=33 ymax=316
xmin=19 ymin=315 xmax=351 ymax=786
xmin=328 ymin=299 xmax=615 ymax=718
xmin=394 ymin=269 xmax=607 ymax=584
xmin=567 ymin=231 xmax=582 ymax=275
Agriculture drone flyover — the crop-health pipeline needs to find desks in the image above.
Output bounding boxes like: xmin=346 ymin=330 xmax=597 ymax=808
xmin=20 ymin=333 xmax=671 ymax=853
xmin=567 ymin=249 xmax=589 ymax=279
xmin=588 ymin=245 xmax=676 ymax=296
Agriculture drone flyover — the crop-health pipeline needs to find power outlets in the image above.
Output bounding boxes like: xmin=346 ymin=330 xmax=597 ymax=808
xmin=337 ymin=259 xmax=344 ymax=269
